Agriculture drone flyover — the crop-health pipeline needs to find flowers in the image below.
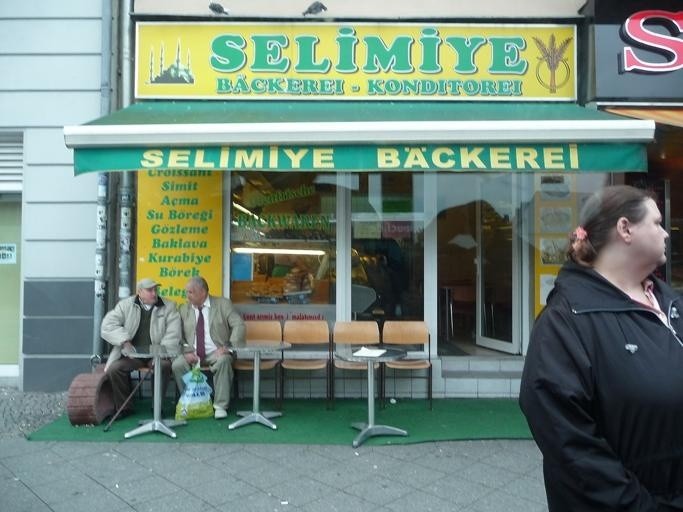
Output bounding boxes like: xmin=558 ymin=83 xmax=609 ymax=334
xmin=283 ymin=268 xmax=314 ymax=292
xmin=245 ymin=273 xmax=286 ymax=298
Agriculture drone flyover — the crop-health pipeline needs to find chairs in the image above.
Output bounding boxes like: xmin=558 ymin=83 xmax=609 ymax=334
xmin=351 ymin=284 xmax=377 ymax=321
xmin=232 ymin=321 xmax=283 ymax=406
xmin=332 ymin=321 xmax=382 ymax=410
xmin=440 ymin=284 xmax=495 ymax=343
xmin=200 ymin=365 xmax=239 ymax=404
xmin=280 ymin=320 xmax=333 ymax=408
xmin=380 ymin=320 xmax=433 ymax=410
xmin=133 ymin=367 xmax=178 ymax=404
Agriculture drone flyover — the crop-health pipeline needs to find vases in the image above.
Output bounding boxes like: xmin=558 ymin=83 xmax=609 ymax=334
xmin=255 ymin=295 xmax=280 ymax=304
xmin=284 ymin=290 xmax=313 ymax=305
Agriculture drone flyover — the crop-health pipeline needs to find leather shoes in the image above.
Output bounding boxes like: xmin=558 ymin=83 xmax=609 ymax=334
xmin=109 ymin=408 xmax=134 ymax=421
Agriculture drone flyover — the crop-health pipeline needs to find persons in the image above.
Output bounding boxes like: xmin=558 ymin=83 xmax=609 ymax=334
xmin=100 ymin=280 xmax=183 ymax=420
xmin=169 ymin=277 xmax=247 ymax=420
xmin=519 ymin=185 xmax=683 ymax=511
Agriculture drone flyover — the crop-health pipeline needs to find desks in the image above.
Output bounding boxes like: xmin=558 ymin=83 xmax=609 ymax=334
xmin=119 ymin=344 xmax=195 ymax=439
xmin=224 ymin=340 xmax=292 ymax=430
xmin=334 ymin=346 xmax=409 ymax=448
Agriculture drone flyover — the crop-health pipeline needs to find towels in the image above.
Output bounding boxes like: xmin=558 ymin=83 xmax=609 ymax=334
xmin=137 ymin=279 xmax=162 ymax=290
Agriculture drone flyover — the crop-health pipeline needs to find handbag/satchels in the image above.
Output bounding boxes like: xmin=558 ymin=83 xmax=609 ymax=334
xmin=175 ymin=357 xmax=214 ymax=420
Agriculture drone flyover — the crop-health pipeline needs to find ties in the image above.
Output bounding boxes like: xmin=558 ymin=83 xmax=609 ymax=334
xmin=196 ymin=308 xmax=205 ymax=363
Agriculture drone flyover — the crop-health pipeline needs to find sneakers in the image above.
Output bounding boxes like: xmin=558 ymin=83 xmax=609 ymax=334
xmin=214 ymin=405 xmax=228 ymax=420
